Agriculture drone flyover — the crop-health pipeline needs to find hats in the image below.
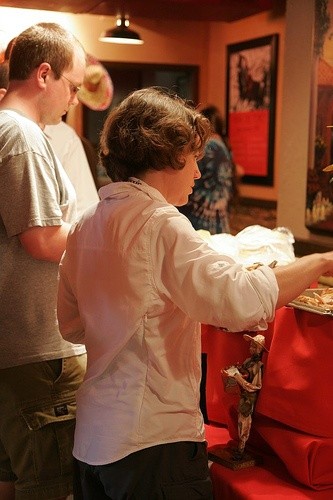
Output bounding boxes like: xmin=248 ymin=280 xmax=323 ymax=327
xmin=77 ymin=53 xmax=114 ymax=112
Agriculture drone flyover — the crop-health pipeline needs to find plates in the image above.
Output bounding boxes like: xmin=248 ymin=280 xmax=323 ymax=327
xmin=287 ymin=287 xmax=333 ymax=316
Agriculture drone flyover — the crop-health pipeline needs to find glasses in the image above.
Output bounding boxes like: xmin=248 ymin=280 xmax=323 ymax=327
xmin=60 ymin=72 xmax=80 ymax=98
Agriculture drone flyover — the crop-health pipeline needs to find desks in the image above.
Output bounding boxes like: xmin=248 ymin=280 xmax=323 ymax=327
xmin=199 ymin=289 xmax=332 ymax=479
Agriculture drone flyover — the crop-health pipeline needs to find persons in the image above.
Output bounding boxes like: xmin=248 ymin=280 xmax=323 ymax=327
xmin=0 ymin=24 xmax=89 ymax=500
xmin=195 ymin=103 xmax=234 ymax=236
xmin=0 ymin=38 xmax=102 ymax=226
xmin=222 ymin=334 xmax=269 ymax=459
xmin=56 ymin=88 xmax=333 ymax=500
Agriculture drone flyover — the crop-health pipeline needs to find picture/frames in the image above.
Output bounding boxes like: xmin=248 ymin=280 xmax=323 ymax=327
xmin=224 ymin=34 xmax=279 ymax=184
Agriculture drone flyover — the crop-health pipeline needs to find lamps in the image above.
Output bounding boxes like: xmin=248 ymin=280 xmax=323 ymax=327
xmin=101 ymin=13 xmax=144 ymax=46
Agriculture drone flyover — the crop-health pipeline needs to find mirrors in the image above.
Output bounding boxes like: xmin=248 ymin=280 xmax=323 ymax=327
xmin=305 ymin=0 xmax=333 ymax=233
